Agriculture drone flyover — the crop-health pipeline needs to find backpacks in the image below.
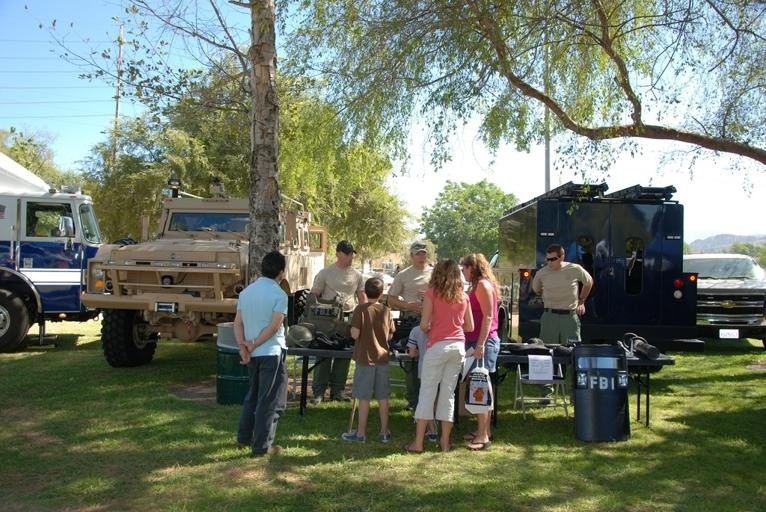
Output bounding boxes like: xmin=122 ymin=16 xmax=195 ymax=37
xmin=300 ymin=292 xmax=344 ymax=338
xmin=385 ymin=309 xmax=422 ymax=354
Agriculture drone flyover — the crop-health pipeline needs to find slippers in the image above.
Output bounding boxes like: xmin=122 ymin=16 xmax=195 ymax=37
xmin=465 ymin=438 xmax=492 ymax=451
xmin=460 ymin=431 xmax=494 ymax=441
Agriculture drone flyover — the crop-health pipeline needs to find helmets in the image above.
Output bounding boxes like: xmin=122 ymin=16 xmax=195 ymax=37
xmin=286 ymin=323 xmax=314 ymax=349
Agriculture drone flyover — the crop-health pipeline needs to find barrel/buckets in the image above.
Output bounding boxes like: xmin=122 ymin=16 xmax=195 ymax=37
xmin=214 ymin=322 xmax=251 ymax=407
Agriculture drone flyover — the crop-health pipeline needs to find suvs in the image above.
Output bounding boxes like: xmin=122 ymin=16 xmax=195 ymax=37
xmin=683 ymin=254 xmax=766 ymax=349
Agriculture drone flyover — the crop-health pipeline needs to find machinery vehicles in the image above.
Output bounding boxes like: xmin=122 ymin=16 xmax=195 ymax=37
xmin=83 ymin=181 xmax=326 ymax=369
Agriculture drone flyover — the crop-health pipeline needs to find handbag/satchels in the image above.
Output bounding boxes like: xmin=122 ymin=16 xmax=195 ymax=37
xmin=464 ymin=353 xmax=496 ymax=415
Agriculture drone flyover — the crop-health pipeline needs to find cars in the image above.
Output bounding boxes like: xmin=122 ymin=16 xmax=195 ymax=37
xmin=428 ymin=264 xmax=472 ymax=292
xmin=366 ymin=270 xmax=394 ymax=286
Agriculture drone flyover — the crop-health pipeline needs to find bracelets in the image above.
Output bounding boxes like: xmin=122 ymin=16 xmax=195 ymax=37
xmin=252 ymin=338 xmax=256 ymax=347
xmin=579 ymin=299 xmax=584 ymax=304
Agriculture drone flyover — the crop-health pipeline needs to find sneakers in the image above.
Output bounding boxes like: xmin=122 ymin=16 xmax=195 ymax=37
xmin=403 ymin=441 xmax=453 ymax=454
xmin=378 ymin=430 xmax=392 ymax=443
xmin=309 ymin=388 xmax=353 ymax=404
xmin=538 ymin=385 xmax=556 ymax=408
xmin=341 ymin=430 xmax=366 ymax=444
xmin=425 ymin=430 xmax=438 ymax=442
xmin=252 ymin=445 xmax=283 ymax=459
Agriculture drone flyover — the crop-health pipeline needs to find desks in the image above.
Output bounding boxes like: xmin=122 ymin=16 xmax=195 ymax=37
xmin=490 ymin=343 xmax=675 ymax=428
xmin=285 ymin=345 xmax=462 ymax=425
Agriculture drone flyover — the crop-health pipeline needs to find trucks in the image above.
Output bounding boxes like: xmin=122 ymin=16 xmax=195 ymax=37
xmin=0 ymin=152 xmax=102 ymax=351
xmin=490 ymin=181 xmax=700 ymax=375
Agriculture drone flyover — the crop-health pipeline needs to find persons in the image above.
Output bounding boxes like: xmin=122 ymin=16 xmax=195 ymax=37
xmin=532 ymin=244 xmax=594 ymax=405
xmin=403 ymin=260 xmax=476 ymax=454
xmin=404 ymin=326 xmax=438 ymax=442
xmin=232 ymin=250 xmax=290 ymax=459
xmin=308 ymin=240 xmax=366 ymax=405
xmin=386 ymin=240 xmax=434 ymax=413
xmin=340 ymin=277 xmax=396 ymax=443
xmin=460 ymin=252 xmax=501 ymax=451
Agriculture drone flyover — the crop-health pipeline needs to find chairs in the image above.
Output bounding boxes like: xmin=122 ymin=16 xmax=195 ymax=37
xmin=292 ymin=355 xmax=325 ymax=402
xmin=513 ymin=363 xmax=570 ymax=422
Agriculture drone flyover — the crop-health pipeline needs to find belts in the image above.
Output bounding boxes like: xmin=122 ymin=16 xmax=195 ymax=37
xmin=543 ymin=308 xmax=575 ymax=315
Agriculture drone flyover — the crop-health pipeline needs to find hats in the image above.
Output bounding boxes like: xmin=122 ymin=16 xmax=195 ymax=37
xmin=336 ymin=240 xmax=358 ymax=255
xmin=408 ymin=240 xmax=428 ymax=255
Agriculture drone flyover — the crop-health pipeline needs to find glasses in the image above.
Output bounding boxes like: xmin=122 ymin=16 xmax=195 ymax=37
xmin=545 ymin=257 xmax=561 ymax=262
